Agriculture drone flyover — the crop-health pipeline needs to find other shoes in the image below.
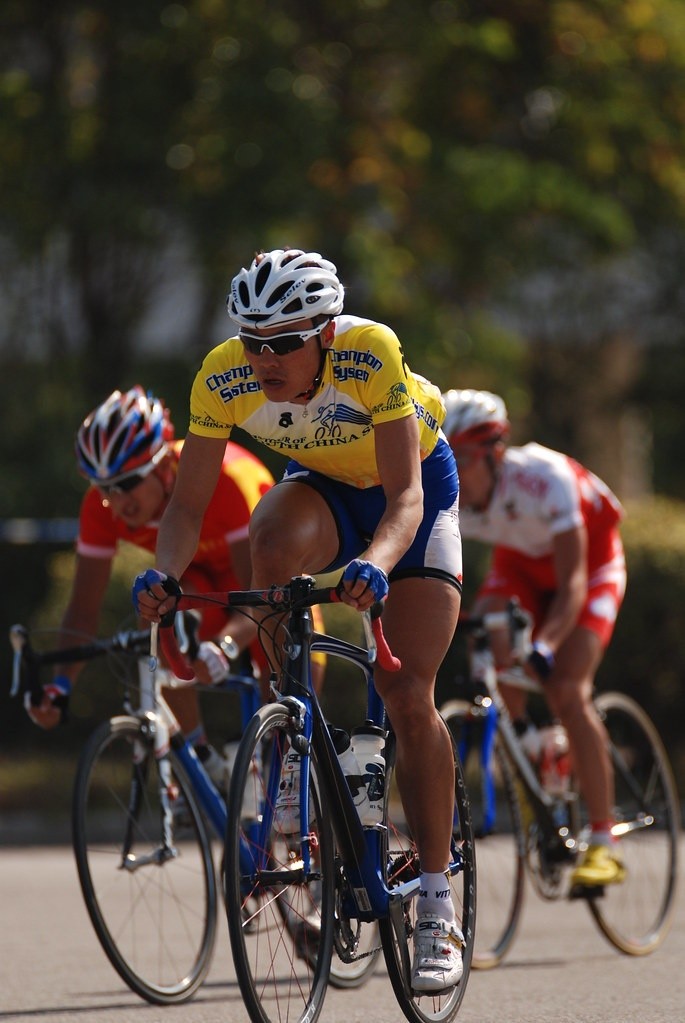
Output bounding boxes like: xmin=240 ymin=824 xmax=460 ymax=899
xmin=464 ymin=721 xmax=543 ymax=778
xmin=571 ymin=830 xmax=626 ymax=885
xmin=172 ymin=742 xmax=233 ymax=819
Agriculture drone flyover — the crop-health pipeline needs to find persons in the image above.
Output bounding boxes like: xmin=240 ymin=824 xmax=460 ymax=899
xmin=130 ymin=247 xmax=466 ymax=992
xmin=22 ymin=381 xmax=326 ymax=938
xmin=439 ymin=385 xmax=629 ymax=888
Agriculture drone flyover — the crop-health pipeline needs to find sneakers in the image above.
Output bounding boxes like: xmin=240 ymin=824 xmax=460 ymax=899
xmin=409 ymin=912 xmax=467 ymax=992
xmin=272 ymin=746 xmax=317 ymax=836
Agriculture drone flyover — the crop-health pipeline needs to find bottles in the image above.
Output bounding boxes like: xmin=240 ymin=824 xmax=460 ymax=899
xmin=350 ymin=718 xmax=387 ymax=826
xmin=325 ymin=722 xmax=370 ymax=818
xmin=513 ymin=719 xmax=541 ymax=761
xmin=536 ymin=714 xmax=571 ymax=796
xmin=195 ymin=743 xmax=229 ymax=788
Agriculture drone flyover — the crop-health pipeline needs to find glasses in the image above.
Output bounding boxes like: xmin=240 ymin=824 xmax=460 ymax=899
xmin=89 ymin=445 xmax=169 ymax=498
xmin=237 ymin=317 xmax=330 ymax=356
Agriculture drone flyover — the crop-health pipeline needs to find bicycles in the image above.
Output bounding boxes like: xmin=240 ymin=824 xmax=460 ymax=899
xmin=5 ymin=604 xmax=384 ymax=1006
xmin=387 ymin=599 xmax=685 ymax=972
xmin=152 ymin=574 xmax=479 ymax=1023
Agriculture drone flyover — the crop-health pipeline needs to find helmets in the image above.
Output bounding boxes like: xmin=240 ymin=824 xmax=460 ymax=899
xmin=73 ymin=385 xmax=174 ymax=484
xmin=227 ymin=249 xmax=345 ymax=329
xmin=440 ymin=389 xmax=509 ymax=459
